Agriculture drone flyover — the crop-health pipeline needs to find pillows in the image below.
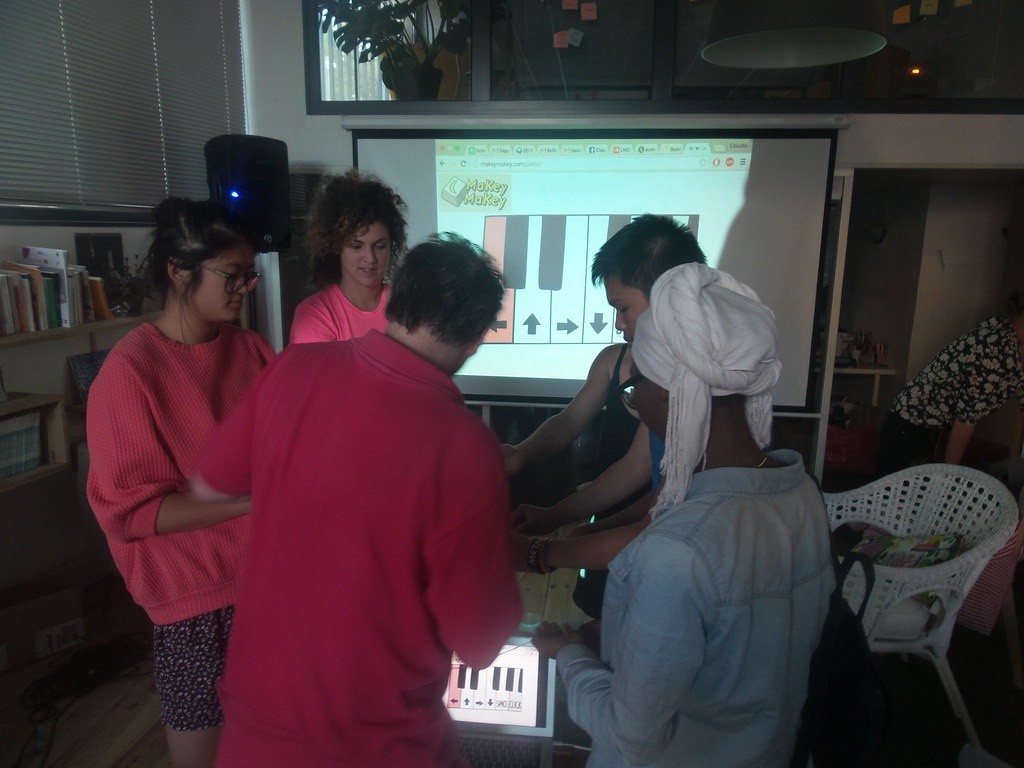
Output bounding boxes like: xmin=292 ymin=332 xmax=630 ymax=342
xmin=851 ymin=533 xmax=960 ymax=608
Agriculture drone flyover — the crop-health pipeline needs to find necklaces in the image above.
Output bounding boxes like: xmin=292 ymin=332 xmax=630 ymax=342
xmin=758 ymin=456 xmax=768 ymax=467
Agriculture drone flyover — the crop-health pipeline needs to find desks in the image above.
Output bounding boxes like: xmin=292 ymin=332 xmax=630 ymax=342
xmin=815 ymin=358 xmax=896 ymax=407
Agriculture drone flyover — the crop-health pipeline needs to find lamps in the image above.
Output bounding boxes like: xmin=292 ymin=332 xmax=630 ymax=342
xmin=699 ymin=0 xmax=888 ymax=70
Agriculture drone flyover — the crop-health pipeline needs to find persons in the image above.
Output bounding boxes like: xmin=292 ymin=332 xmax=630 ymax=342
xmin=84 ymin=200 xmax=275 ymax=768
xmin=288 ymin=171 xmax=407 ymax=344
xmin=566 ymin=211 xmax=707 ymax=535
xmin=878 ymin=312 xmax=1024 ymax=476
xmin=495 ymin=339 xmax=648 ymax=618
xmin=191 ymin=240 xmax=523 ymax=768
xmin=528 ymin=261 xmax=836 ymax=768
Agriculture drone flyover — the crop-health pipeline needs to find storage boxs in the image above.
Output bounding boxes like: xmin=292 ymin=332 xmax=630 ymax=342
xmin=783 ymin=420 xmax=876 ymax=472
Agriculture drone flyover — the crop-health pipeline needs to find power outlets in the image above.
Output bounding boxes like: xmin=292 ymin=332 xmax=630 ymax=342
xmin=33 ymin=617 xmax=86 ymax=658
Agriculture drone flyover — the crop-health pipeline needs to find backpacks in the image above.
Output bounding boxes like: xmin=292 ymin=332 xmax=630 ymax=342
xmin=791 ymin=471 xmax=884 ymax=768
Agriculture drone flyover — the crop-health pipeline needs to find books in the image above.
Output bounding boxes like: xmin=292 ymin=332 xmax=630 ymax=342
xmin=0 ymin=245 xmax=114 ymax=335
xmin=0 ymin=410 xmax=42 ymax=479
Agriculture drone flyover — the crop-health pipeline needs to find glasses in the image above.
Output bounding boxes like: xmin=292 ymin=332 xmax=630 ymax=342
xmin=197 ymin=265 xmax=260 ymax=294
xmin=617 ymin=374 xmax=643 ymax=420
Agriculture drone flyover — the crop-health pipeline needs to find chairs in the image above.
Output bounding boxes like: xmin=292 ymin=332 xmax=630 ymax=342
xmin=878 ymin=509 xmax=1024 ymax=636
xmin=823 ymin=463 xmax=1020 ymax=748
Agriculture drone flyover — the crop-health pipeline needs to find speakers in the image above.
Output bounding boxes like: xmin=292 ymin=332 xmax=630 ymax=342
xmin=204 ymin=134 xmax=293 ymax=253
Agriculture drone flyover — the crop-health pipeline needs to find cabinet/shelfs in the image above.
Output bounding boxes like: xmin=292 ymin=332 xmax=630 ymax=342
xmin=0 ymin=295 xmax=259 ymax=495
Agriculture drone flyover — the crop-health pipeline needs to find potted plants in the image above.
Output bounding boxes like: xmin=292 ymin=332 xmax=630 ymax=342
xmin=317 ymin=0 xmax=506 ymax=101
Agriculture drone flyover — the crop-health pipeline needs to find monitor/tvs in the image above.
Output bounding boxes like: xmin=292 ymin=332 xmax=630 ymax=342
xmin=441 ymin=637 xmax=557 ymax=738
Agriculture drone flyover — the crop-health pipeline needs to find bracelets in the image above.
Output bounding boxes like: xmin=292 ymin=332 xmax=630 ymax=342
xmin=529 ymin=537 xmax=559 ymax=574
